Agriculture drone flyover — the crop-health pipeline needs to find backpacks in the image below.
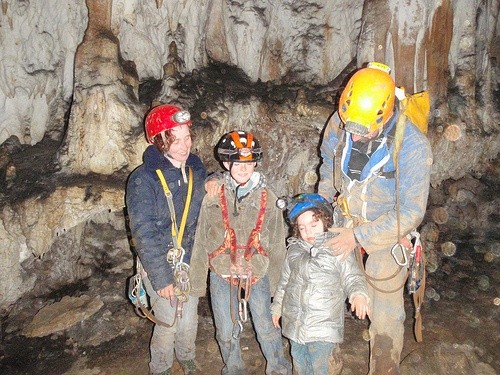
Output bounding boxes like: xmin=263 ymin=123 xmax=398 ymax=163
xmin=334 ymin=87 xmax=430 ymax=191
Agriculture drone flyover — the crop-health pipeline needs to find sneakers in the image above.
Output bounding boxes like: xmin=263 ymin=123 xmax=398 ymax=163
xmin=151 ymin=359 xmax=203 ymax=375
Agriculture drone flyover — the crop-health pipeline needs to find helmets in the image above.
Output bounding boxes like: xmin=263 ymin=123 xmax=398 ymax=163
xmin=145 ymin=105 xmax=193 ymax=143
xmin=287 ymin=194 xmax=334 ymax=222
xmin=339 ymin=62 xmax=396 ymax=136
xmin=218 ymin=131 xmax=263 ymax=162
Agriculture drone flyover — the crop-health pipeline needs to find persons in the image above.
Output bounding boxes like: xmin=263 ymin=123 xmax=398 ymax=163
xmin=188 ymin=129 xmax=289 ymax=375
xmin=318 ymin=61 xmax=435 ymax=374
xmin=125 ymin=104 xmax=207 ymax=375
xmin=270 ymin=192 xmax=371 ymax=375
